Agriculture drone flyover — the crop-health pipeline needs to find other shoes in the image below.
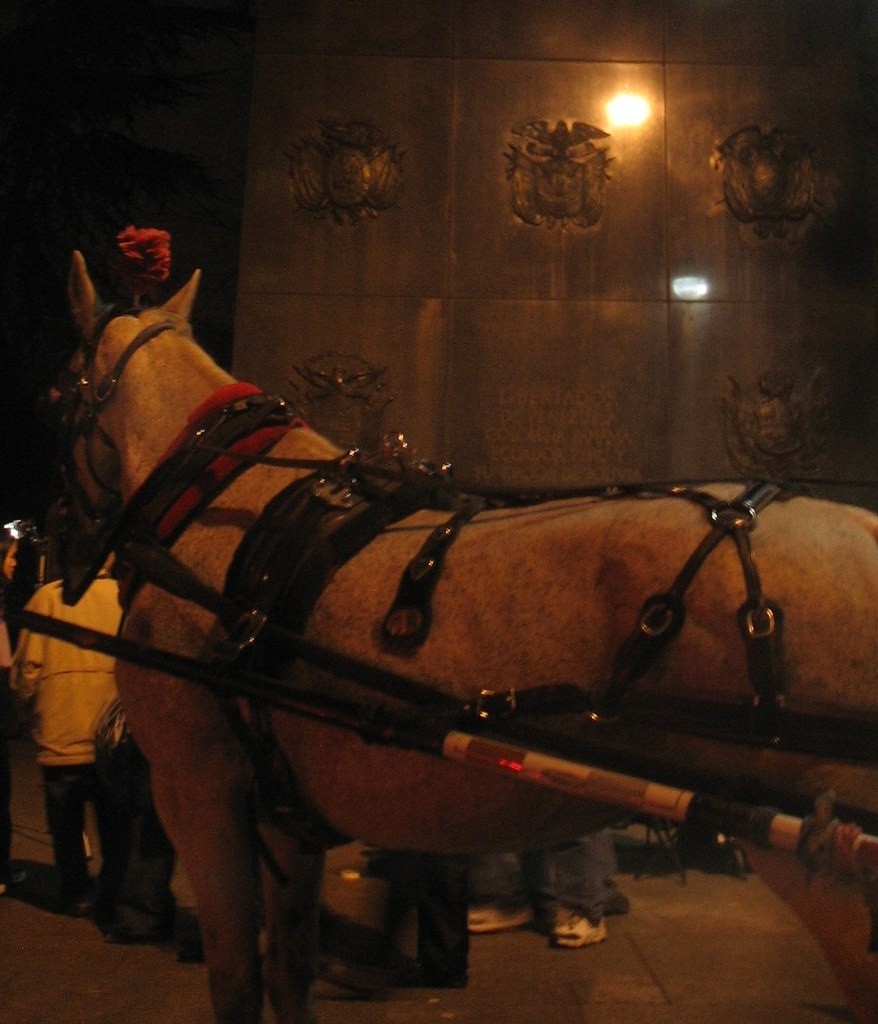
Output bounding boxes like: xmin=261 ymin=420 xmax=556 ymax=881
xmin=109 ymin=914 xmax=162 ymax=944
xmin=171 ymin=934 xmax=203 ymax=963
xmin=380 ymin=959 xmax=468 ymax=988
xmin=550 ymin=913 xmax=607 ymax=947
xmin=464 ymin=899 xmax=538 ymax=935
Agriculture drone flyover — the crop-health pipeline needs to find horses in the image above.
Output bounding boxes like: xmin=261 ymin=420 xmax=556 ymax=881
xmin=45 ymin=248 xmax=878 ymax=1024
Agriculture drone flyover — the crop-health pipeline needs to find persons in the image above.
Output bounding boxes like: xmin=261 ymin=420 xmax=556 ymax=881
xmin=465 ymin=832 xmax=607 ymax=951
xmin=0 ymin=533 xmax=204 ymax=962
